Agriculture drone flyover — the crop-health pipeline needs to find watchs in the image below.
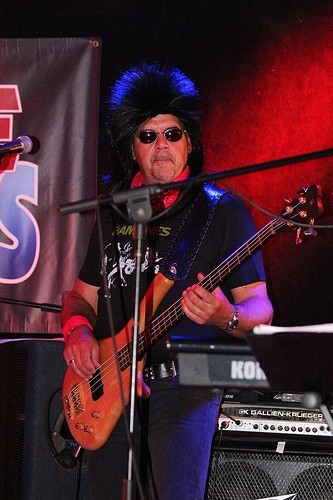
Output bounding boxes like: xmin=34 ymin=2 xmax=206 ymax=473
xmin=224 ymin=305 xmax=238 ymax=331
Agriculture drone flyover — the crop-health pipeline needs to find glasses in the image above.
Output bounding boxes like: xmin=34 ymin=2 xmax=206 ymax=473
xmin=133 ymin=126 xmax=186 ymax=145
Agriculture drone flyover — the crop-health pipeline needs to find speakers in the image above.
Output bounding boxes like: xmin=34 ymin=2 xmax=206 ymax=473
xmin=0 ymin=335 xmax=132 ymax=500
xmin=203 ymin=444 xmax=333 ymax=500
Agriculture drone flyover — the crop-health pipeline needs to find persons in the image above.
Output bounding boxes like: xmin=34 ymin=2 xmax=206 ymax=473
xmin=61 ymin=64 xmax=272 ymax=500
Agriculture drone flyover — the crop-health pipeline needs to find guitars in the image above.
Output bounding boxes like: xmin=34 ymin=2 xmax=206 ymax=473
xmin=61 ymin=182 xmax=323 ymax=450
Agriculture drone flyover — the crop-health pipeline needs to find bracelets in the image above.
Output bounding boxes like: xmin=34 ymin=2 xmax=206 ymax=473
xmin=62 ymin=315 xmax=93 ymax=343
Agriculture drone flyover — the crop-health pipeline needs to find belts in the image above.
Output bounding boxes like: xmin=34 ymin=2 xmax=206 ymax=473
xmin=141 ymin=360 xmax=178 ymax=384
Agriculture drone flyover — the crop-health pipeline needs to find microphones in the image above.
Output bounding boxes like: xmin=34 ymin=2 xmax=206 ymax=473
xmin=0 ymin=135 xmax=33 ymax=156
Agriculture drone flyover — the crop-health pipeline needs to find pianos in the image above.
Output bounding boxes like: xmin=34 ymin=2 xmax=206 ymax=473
xmin=144 ymin=342 xmax=271 ymax=390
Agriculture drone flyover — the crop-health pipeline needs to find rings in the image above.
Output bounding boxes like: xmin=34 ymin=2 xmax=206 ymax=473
xmin=69 ymin=360 xmax=74 ymax=363
